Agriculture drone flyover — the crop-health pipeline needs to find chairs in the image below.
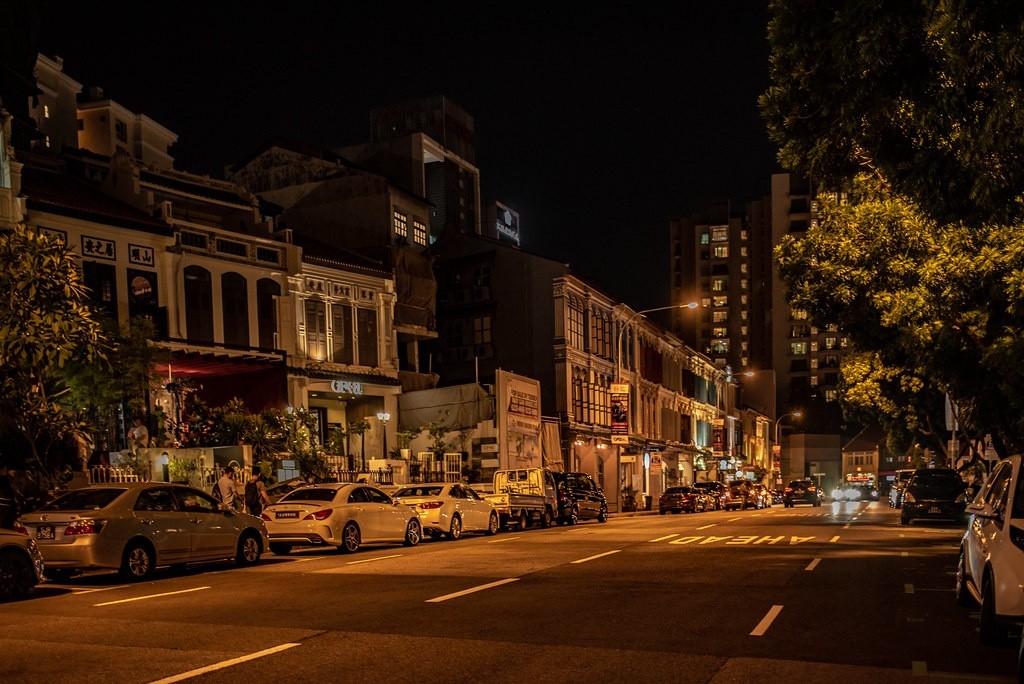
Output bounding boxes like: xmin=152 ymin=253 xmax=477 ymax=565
xmin=423 ymin=457 xmax=445 ymax=482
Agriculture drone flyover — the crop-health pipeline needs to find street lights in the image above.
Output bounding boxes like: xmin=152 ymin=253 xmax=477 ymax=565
xmin=716 ymin=371 xmax=756 ymax=481
xmin=287 ymin=406 xmax=305 ymax=456
xmin=777 ymin=413 xmax=801 ymax=491
xmin=616 ymin=302 xmax=699 ymax=513
xmin=378 ymin=410 xmax=390 ymax=459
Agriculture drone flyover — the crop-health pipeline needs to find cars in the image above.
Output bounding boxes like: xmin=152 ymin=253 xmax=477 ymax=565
xmin=0 ymin=527 xmax=44 ymax=601
xmin=12 ymin=481 xmax=269 ymax=582
xmin=901 ymin=469 xmax=970 ymax=526
xmin=263 ymin=482 xmax=426 ymax=553
xmin=723 ymin=479 xmax=772 ymax=510
xmin=769 ymin=490 xmax=784 ymax=505
xmin=831 ymin=485 xmax=879 ymax=501
xmin=784 ymin=479 xmax=822 ymax=507
xmin=392 ymin=483 xmax=501 ymax=539
xmin=698 ymin=489 xmax=717 ymax=511
xmin=955 ymin=454 xmax=1024 ymax=635
xmin=659 ymin=486 xmax=708 ymax=513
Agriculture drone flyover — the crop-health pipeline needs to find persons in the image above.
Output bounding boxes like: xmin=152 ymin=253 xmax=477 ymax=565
xmin=242 ymin=467 xmax=271 ymax=516
xmin=216 ymin=467 xmax=240 ymax=510
xmin=127 ymin=416 xmax=148 ymax=456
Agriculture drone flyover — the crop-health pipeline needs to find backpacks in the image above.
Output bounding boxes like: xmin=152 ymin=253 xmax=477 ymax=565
xmin=244 ymin=480 xmax=262 ymax=506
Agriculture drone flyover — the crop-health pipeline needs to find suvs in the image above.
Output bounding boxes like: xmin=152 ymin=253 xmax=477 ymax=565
xmin=695 ymin=481 xmax=727 ymax=510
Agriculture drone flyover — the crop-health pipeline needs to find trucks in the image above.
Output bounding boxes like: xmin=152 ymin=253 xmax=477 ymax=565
xmin=476 ymin=465 xmax=565 ymax=531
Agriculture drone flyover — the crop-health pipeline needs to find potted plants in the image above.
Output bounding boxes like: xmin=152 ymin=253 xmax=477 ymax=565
xmin=458 ymin=429 xmax=474 ymax=462
xmin=426 ymin=421 xmax=448 ymax=461
xmin=396 ymin=431 xmax=419 ymax=460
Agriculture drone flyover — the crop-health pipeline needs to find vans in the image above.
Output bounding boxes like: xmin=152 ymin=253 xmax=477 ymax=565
xmin=888 ymin=470 xmax=916 ymax=507
xmin=546 ymin=471 xmax=609 ymax=525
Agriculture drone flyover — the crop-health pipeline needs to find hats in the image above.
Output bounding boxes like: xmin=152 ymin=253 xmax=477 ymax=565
xmin=223 ymin=467 xmax=234 ymax=473
xmin=252 ymin=467 xmax=261 ymax=473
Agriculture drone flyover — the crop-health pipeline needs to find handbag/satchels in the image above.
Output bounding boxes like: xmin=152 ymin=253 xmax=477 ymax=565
xmin=212 ymin=479 xmax=222 ymax=502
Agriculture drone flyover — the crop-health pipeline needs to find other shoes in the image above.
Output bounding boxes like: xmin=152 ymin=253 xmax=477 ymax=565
xmin=127 ymin=452 xmax=136 ymax=458
